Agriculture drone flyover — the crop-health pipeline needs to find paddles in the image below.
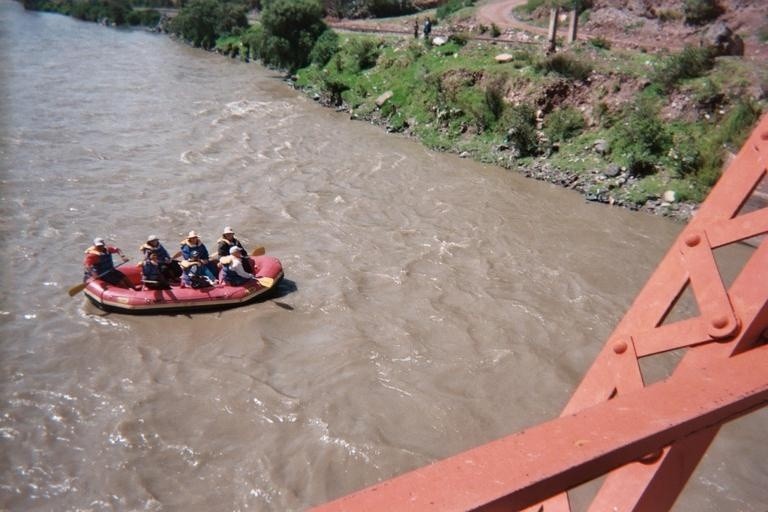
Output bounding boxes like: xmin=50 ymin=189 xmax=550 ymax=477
xmin=171 ymin=252 xmax=181 ymax=261
xmin=252 ymin=276 xmax=275 ymax=288
xmin=68 ymin=260 xmax=130 ymax=297
xmin=241 ymin=247 xmax=266 ymax=257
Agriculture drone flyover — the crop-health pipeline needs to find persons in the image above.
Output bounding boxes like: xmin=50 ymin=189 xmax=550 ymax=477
xmin=82 ymin=236 xmax=140 ymax=293
xmin=412 ymin=16 xmax=420 ymax=38
xmin=423 ymin=16 xmax=433 ymax=40
xmin=140 ymin=226 xmax=259 ymax=290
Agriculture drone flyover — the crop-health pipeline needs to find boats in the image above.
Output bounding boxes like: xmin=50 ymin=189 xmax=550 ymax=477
xmin=79 ymin=253 xmax=284 ymax=313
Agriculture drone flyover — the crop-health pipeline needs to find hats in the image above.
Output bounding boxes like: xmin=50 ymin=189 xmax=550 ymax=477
xmin=186 ymin=230 xmax=200 ymax=239
xmin=92 ymin=237 xmax=105 ymax=247
xmin=190 ymin=250 xmax=200 ymax=259
xmin=222 ymin=226 xmax=235 ymax=237
xmin=147 ymin=234 xmax=160 ymax=244
xmin=229 ymin=245 xmax=243 ymax=255
